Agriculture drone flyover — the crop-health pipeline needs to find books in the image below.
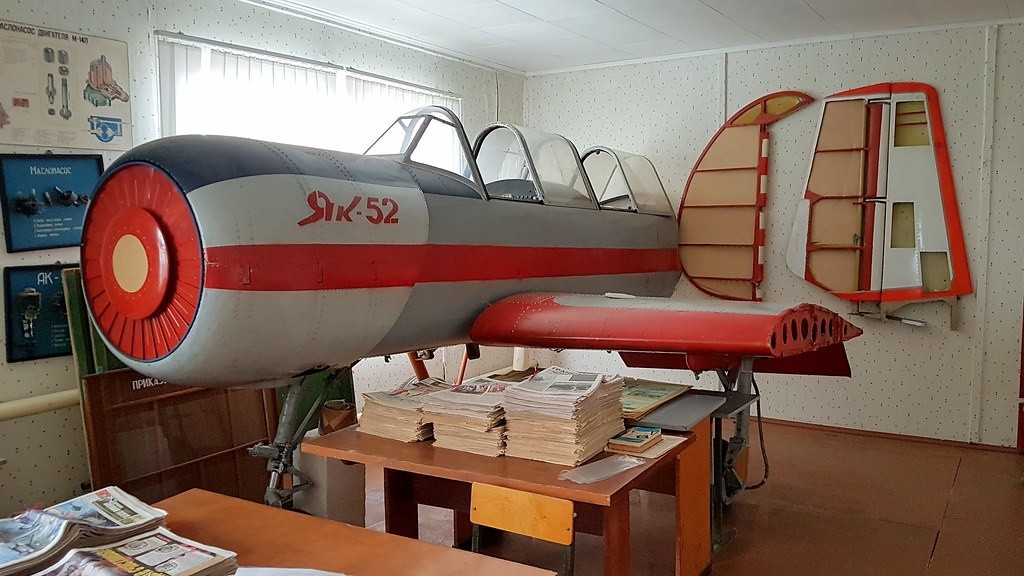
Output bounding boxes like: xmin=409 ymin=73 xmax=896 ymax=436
xmin=607 ymin=426 xmax=663 ymax=452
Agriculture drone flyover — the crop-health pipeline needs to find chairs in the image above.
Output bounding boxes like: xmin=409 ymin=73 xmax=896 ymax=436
xmin=469 ymin=482 xmax=577 ymax=576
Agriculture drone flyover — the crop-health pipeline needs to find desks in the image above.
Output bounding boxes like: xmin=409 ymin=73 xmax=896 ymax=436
xmin=302 ymin=370 xmax=760 ymax=576
xmin=150 ymin=489 xmax=559 ymax=576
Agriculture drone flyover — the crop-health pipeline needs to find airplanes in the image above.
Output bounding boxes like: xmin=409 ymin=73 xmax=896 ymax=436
xmin=76 ymin=100 xmax=864 ymax=514
